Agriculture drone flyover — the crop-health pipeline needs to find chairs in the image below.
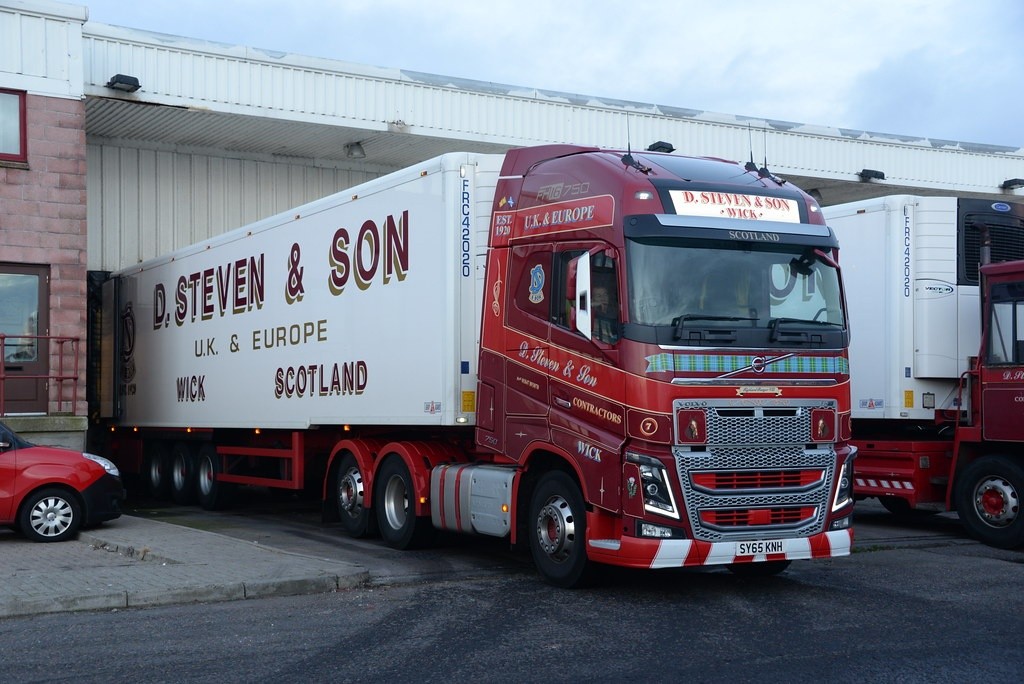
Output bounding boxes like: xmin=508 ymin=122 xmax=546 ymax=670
xmin=699 ymin=272 xmax=740 ymax=313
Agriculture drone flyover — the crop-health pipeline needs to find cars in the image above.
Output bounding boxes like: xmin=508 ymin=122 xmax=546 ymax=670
xmin=0 ymin=419 xmax=128 ymax=544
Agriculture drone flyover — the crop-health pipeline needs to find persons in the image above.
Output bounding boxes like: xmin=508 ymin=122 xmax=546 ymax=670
xmin=591 ymin=282 xmax=618 ymax=345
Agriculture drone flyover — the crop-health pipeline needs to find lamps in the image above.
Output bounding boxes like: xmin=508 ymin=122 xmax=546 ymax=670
xmin=347 ymin=144 xmax=366 ymax=158
xmin=107 ymin=74 xmax=142 ymax=93
xmin=1000 ymin=178 xmax=1024 ymax=190
xmin=859 ymin=169 xmax=887 ymax=180
xmin=647 ymin=141 xmax=677 ymax=153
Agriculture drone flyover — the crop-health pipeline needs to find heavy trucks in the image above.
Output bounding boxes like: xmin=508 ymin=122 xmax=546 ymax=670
xmin=763 ymin=192 xmax=1024 ymax=551
xmin=92 ymin=140 xmax=858 ymax=591
